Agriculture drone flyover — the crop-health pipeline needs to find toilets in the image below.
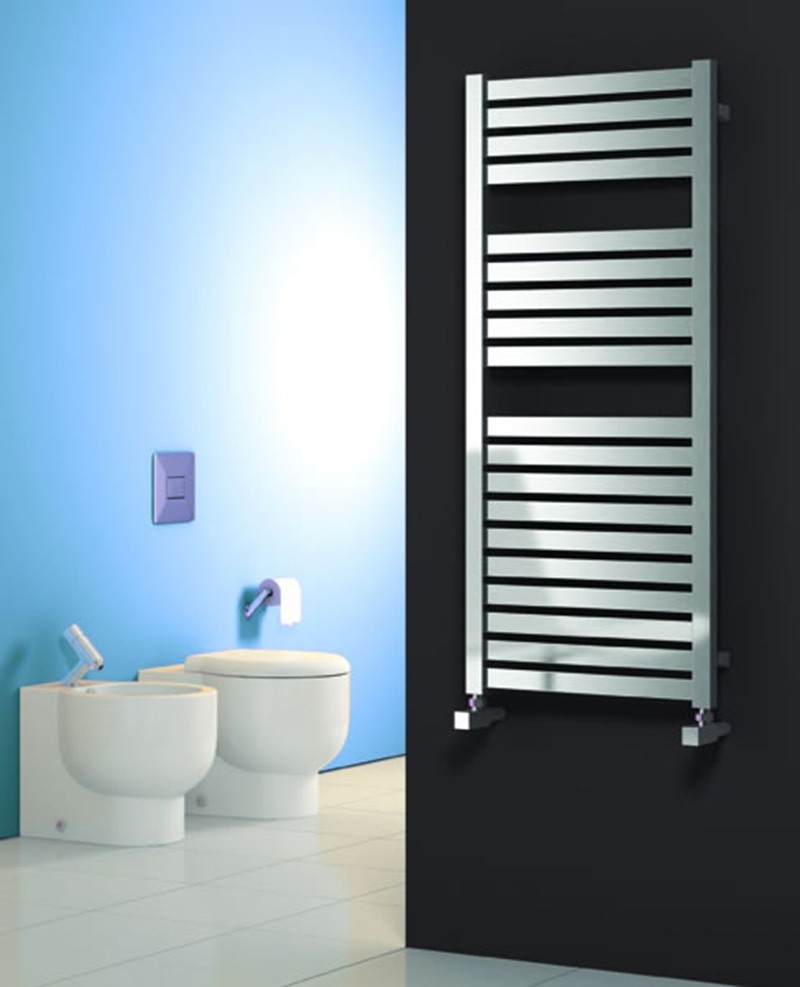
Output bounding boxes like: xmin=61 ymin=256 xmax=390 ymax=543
xmin=136 ymin=650 xmax=353 ymax=820
xmin=18 ymin=670 xmax=220 ymax=849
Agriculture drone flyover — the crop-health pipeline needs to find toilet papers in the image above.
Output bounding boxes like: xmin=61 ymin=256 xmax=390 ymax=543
xmin=260 ymin=575 xmax=304 ymax=626
